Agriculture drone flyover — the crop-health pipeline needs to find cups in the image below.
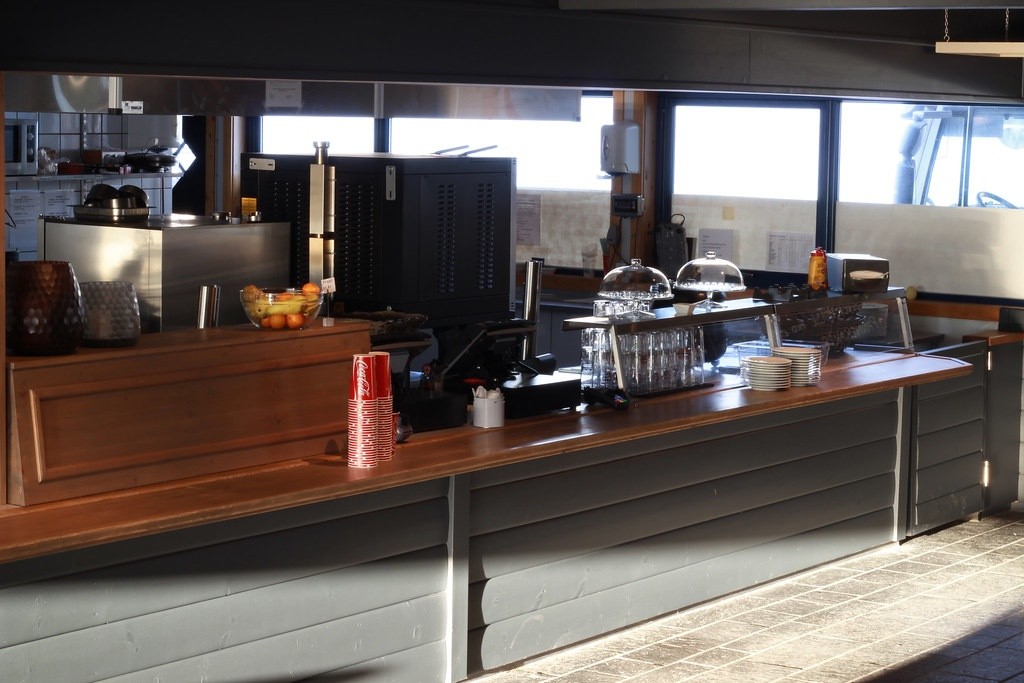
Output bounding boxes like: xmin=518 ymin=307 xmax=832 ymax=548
xmin=347 ymin=351 xmax=392 ymax=468
xmin=582 ymin=250 xmax=597 ymax=277
xmin=392 ymin=409 xmax=400 ymax=456
xmin=81 ymin=282 xmax=140 ymax=346
xmin=4 ymin=260 xmax=80 ymax=357
xmin=474 ymin=398 xmax=504 ymax=428
xmin=581 ymin=327 xmax=705 ymax=391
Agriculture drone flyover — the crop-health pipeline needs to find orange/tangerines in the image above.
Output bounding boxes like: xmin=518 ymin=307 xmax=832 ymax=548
xmin=259 ymin=283 xmax=320 ymax=329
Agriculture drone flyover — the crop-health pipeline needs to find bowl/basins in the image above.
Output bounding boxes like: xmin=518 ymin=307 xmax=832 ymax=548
xmin=673 ymin=303 xmax=697 ymax=313
xmin=542 ymin=268 xmax=556 ymax=274
xmin=240 ymin=289 xmax=323 ymax=329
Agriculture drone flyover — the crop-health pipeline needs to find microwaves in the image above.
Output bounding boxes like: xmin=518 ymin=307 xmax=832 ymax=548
xmin=5 ymin=119 xmax=39 ymax=175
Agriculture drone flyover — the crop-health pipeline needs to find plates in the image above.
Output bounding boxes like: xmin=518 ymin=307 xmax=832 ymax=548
xmin=740 ymin=347 xmax=822 ymax=391
xmin=854 ymin=303 xmax=889 ymax=338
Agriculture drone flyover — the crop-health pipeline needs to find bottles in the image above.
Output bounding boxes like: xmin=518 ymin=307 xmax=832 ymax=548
xmin=807 ymin=247 xmax=826 ymax=290
